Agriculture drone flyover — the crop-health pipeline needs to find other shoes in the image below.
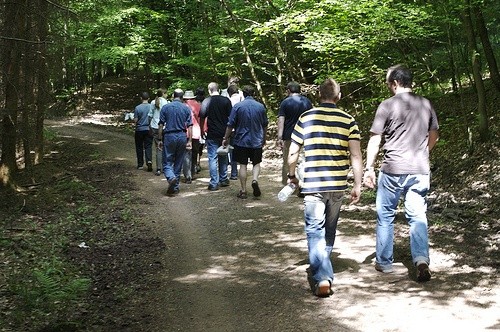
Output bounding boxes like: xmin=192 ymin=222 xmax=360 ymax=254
xmin=208 ymin=184 xmax=219 ymax=191
xmin=196 ymin=165 xmax=201 ymax=172
xmin=167 ymin=178 xmax=178 ymax=195
xmin=375 ymin=265 xmax=392 ymax=273
xmin=416 ymin=259 xmax=431 ymax=282
xmin=297 ymin=186 xmax=305 ymax=199
xmin=192 ymin=174 xmax=196 ymax=180
xmin=184 ymin=176 xmax=192 ymax=184
xmin=316 ymin=279 xmax=331 ymax=296
xmin=147 ymin=161 xmax=152 ymax=172
xmin=155 ymin=170 xmax=160 ymax=176
xmin=229 ymin=174 xmax=238 ymax=180
xmin=220 ymin=179 xmax=229 ymax=186
xmin=137 ymin=164 xmax=143 ymax=169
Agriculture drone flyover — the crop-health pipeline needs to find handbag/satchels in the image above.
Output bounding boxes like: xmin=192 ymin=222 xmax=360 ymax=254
xmin=215 ymin=138 xmax=234 ymax=156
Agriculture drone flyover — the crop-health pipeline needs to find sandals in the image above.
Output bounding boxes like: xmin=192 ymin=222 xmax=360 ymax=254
xmin=252 ymin=180 xmax=261 ymax=197
xmin=237 ymin=190 xmax=247 ymax=199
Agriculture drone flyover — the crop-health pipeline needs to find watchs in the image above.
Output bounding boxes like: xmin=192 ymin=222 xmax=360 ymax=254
xmin=287 ymin=172 xmax=295 ymax=179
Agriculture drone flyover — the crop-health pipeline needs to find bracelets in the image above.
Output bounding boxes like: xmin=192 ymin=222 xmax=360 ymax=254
xmin=365 ymin=167 xmax=375 ymax=171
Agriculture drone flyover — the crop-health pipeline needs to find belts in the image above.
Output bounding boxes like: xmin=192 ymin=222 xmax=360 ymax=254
xmin=163 ymin=130 xmax=188 ymax=133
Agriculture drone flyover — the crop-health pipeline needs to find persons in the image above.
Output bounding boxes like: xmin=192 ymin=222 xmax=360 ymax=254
xmin=363 ymin=63 xmax=439 ymax=282
xmin=134 ymin=82 xmax=269 ymax=199
xmin=287 ymin=78 xmax=363 ymax=296
xmin=277 ymin=81 xmax=313 ymax=197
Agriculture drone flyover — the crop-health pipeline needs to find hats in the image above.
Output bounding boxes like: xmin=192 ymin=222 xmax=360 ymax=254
xmin=182 ymin=90 xmax=196 ymax=99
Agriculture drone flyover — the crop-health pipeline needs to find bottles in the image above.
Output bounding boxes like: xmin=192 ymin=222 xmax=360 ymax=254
xmin=278 ymin=182 xmax=296 ymax=202
